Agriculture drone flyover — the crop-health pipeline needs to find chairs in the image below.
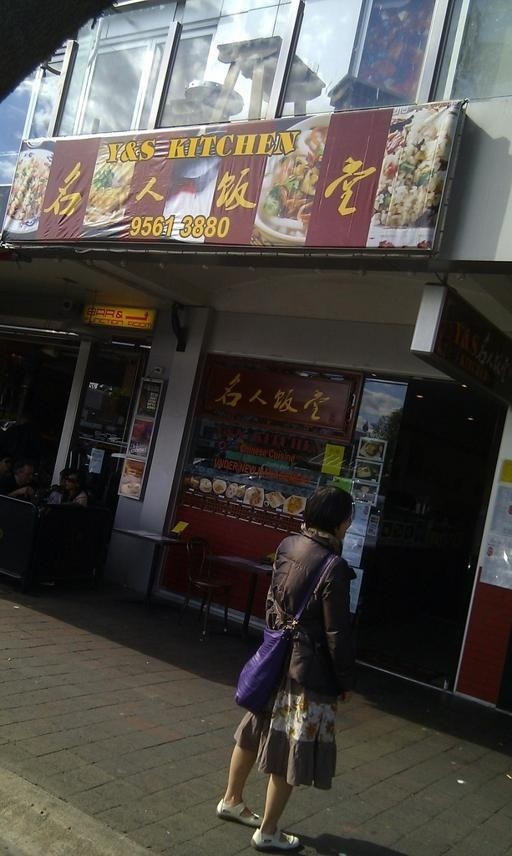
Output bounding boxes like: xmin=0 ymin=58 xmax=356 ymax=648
xmin=176 ymin=535 xmax=230 ymax=642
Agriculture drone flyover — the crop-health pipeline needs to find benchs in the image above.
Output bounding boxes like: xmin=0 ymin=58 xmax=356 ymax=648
xmin=328 ymin=74 xmax=418 ymax=110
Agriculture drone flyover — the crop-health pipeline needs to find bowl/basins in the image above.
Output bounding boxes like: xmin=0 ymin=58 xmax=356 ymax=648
xmin=257 ymin=116 xmax=330 ymax=246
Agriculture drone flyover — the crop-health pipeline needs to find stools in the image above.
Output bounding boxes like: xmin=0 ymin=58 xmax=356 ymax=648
xmin=185 ymin=79 xmax=245 ymax=124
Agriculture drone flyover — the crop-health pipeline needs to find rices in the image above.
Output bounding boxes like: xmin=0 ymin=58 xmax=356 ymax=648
xmin=370 ymin=109 xmax=456 ymax=228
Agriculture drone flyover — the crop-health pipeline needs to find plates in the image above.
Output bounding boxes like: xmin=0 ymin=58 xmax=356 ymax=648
xmin=7 ymin=148 xmax=52 ymax=236
xmin=200 ymin=479 xmax=306 ymax=517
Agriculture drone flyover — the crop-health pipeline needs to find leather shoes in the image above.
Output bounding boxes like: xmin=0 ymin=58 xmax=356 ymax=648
xmin=215 ymin=798 xmax=263 ymax=828
xmin=251 ymin=828 xmax=300 ymax=851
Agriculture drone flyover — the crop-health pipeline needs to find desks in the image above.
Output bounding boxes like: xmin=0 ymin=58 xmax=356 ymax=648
xmin=208 ymin=553 xmax=274 ymax=642
xmin=208 ymin=36 xmax=326 ymax=121
xmin=111 ymin=521 xmax=206 ymax=614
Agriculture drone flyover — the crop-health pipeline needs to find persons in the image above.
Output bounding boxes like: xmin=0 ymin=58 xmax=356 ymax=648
xmin=1 ymin=456 xmax=89 ymax=508
xmin=215 ymin=486 xmax=355 ymax=850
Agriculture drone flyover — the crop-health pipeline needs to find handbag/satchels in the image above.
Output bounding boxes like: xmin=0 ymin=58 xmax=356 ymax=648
xmin=235 ymin=626 xmax=295 ymax=715
